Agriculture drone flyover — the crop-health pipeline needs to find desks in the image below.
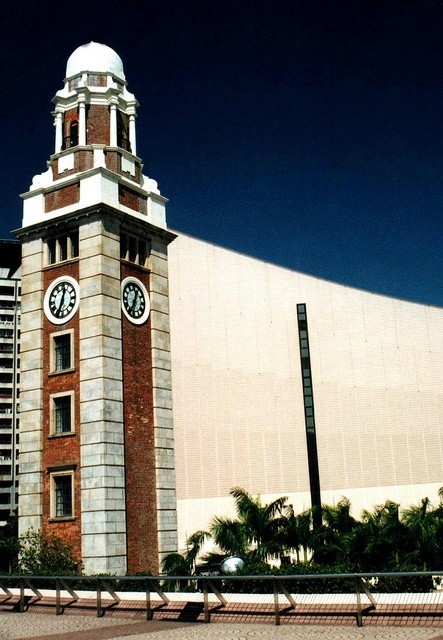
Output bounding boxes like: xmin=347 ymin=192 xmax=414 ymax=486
xmin=296 ymin=302 xmax=323 ymax=566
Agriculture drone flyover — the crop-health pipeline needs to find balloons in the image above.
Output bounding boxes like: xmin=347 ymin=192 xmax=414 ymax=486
xmin=43 ymin=275 xmax=80 ymax=325
xmin=121 ymin=276 xmax=150 ymax=325
